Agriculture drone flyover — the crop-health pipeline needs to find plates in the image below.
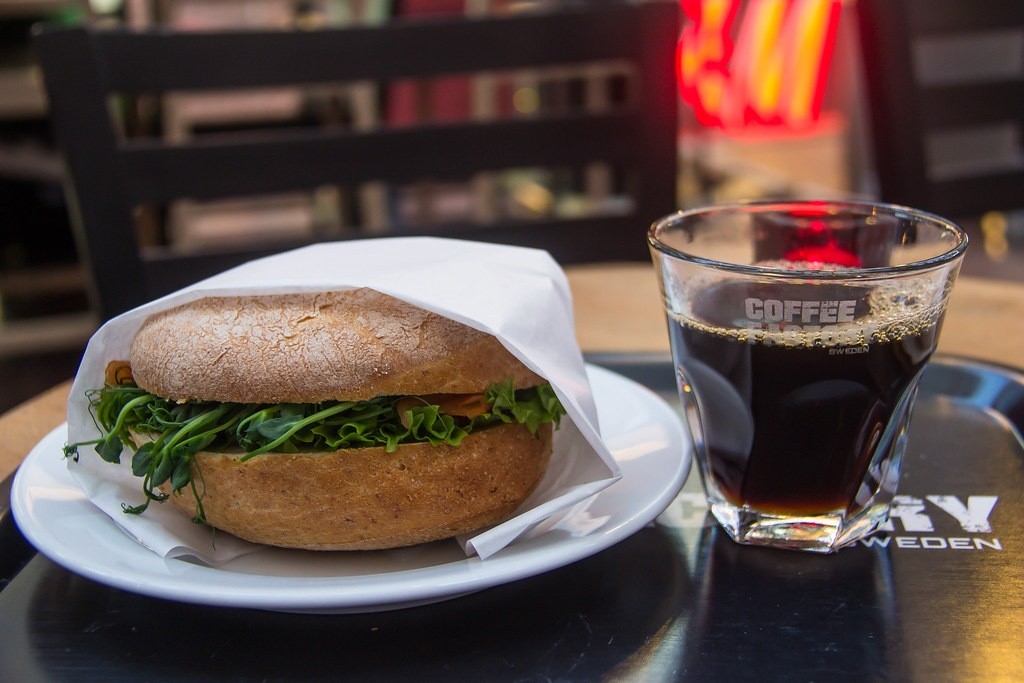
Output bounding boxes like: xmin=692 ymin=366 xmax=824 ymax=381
xmin=9 ymin=363 xmax=694 ymax=614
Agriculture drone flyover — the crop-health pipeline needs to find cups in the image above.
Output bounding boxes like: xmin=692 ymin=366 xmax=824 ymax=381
xmin=647 ymin=198 xmax=970 ymax=553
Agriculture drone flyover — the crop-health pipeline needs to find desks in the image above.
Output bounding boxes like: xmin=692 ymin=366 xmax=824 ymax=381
xmin=0 ymin=262 xmax=1024 ymax=474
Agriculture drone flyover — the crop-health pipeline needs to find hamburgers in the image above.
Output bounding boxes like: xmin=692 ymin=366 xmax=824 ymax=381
xmin=92 ymin=287 xmax=563 ymax=550
